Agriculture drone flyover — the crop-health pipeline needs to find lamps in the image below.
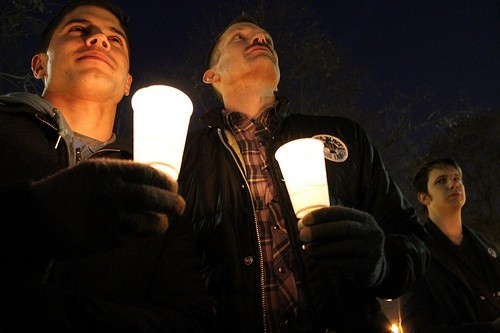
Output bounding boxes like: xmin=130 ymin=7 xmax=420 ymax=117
xmin=130 ymin=83 xmax=195 ymax=181
xmin=274 ymin=138 xmax=331 ymax=218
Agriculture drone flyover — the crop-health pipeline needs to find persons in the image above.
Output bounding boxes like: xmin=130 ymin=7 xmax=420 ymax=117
xmin=1 ymin=1 xmax=186 ymax=333
xmin=162 ymin=13 xmax=432 ymax=333
xmin=397 ymin=156 xmax=500 ymax=333
